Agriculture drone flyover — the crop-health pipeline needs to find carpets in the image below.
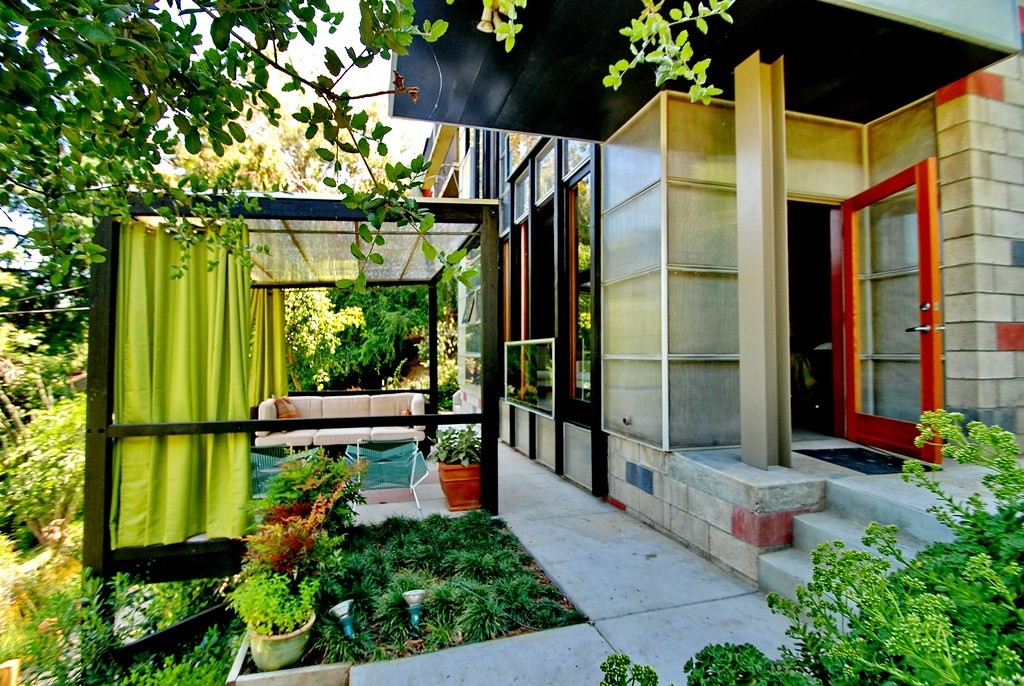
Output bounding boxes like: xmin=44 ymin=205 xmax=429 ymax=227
xmin=792 ymin=448 xmax=943 ymax=475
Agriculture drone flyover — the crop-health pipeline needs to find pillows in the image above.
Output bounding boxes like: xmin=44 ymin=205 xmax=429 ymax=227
xmin=272 ymin=394 xmax=297 ymax=434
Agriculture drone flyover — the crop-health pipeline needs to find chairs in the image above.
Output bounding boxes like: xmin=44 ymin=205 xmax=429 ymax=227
xmin=249 ymin=446 xmax=320 ymax=500
xmin=342 ymin=437 xmax=430 ymax=512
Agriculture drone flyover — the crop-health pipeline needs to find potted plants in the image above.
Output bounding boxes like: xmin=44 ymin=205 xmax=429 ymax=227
xmin=230 ymin=573 xmax=316 ymax=673
xmin=427 ymin=425 xmax=481 ymax=511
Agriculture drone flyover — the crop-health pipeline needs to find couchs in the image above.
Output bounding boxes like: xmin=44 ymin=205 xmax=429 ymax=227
xmin=254 ymin=393 xmax=427 ymax=463
